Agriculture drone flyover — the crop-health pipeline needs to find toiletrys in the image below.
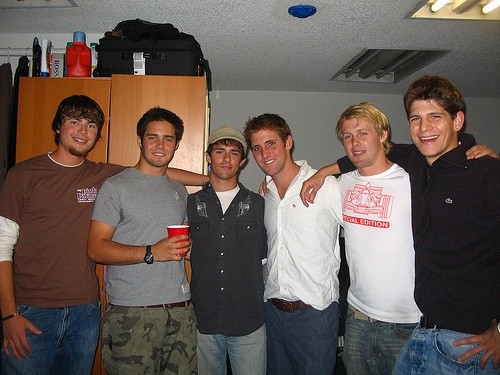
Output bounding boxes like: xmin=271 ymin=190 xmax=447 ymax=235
xmin=39 ymin=38 xmax=52 ymax=77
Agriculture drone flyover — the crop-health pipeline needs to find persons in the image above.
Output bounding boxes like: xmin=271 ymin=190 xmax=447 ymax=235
xmin=243 ymin=113 xmax=344 ymax=375
xmin=185 ymin=126 xmax=269 ymax=375
xmin=299 ymin=74 xmax=500 ymax=375
xmin=0 ymin=94 xmax=209 ymax=375
xmin=259 ymin=102 xmax=423 ymax=375
xmin=87 ymin=106 xmax=198 ymax=375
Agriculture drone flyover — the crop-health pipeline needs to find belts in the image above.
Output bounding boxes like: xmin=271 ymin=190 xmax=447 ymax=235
xmin=271 ymin=298 xmax=312 ymax=312
xmin=139 ymin=300 xmax=189 ymax=309
xmin=420 ymin=315 xmax=488 ymax=335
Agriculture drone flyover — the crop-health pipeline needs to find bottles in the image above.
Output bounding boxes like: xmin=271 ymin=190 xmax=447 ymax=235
xmin=64 ymin=31 xmax=99 ymax=77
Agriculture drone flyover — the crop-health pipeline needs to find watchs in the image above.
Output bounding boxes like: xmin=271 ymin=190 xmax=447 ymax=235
xmin=143 ymin=245 xmax=153 ymax=265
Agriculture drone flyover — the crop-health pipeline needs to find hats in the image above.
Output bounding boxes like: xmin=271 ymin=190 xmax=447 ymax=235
xmin=207 ymin=126 xmax=248 ymax=154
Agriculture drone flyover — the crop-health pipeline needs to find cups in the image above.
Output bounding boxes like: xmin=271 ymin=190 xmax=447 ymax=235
xmin=167 ymin=225 xmax=190 ymax=257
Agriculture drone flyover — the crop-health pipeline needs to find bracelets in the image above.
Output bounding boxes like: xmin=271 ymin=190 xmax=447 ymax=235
xmin=1 ymin=311 xmax=20 ymax=322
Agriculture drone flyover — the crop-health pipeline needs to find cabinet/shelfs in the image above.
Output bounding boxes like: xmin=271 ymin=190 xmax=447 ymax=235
xmin=15 ymin=73 xmax=211 ymax=195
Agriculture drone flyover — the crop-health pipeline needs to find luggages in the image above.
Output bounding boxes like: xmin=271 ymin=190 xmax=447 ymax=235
xmin=92 ymin=35 xmax=204 ymax=77
